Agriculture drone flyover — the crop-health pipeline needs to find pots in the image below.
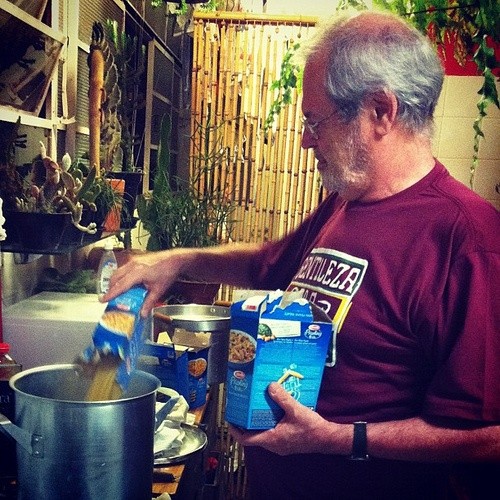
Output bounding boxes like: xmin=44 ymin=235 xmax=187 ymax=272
xmin=152 ymin=301 xmax=231 ymax=386
xmin=0 ymin=364 xmax=180 ymax=500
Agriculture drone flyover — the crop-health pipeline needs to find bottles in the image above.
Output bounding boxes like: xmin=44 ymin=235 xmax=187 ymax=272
xmin=0 ymin=343 xmax=22 ymax=423
xmin=96 ymin=239 xmax=118 ymax=303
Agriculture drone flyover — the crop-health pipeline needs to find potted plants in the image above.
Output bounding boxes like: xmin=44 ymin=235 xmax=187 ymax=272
xmin=166 ymin=144 xmax=240 ymax=306
xmin=3 ymin=135 xmax=144 ymax=246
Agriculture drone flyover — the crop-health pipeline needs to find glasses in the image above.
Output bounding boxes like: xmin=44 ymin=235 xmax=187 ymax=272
xmin=299 ymin=98 xmax=354 ymax=140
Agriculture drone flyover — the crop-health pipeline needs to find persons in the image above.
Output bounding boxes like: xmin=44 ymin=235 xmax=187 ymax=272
xmin=97 ymin=13 xmax=500 ymax=500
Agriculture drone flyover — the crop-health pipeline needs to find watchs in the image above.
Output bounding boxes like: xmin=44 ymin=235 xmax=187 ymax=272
xmin=351 ymin=422 xmax=369 ymax=462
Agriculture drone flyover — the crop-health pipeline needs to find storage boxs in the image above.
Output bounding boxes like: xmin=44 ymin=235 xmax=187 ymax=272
xmin=146 ymin=328 xmax=213 ymax=410
xmin=225 ymin=289 xmax=333 ymax=430
xmin=74 ymin=285 xmax=148 ymax=390
